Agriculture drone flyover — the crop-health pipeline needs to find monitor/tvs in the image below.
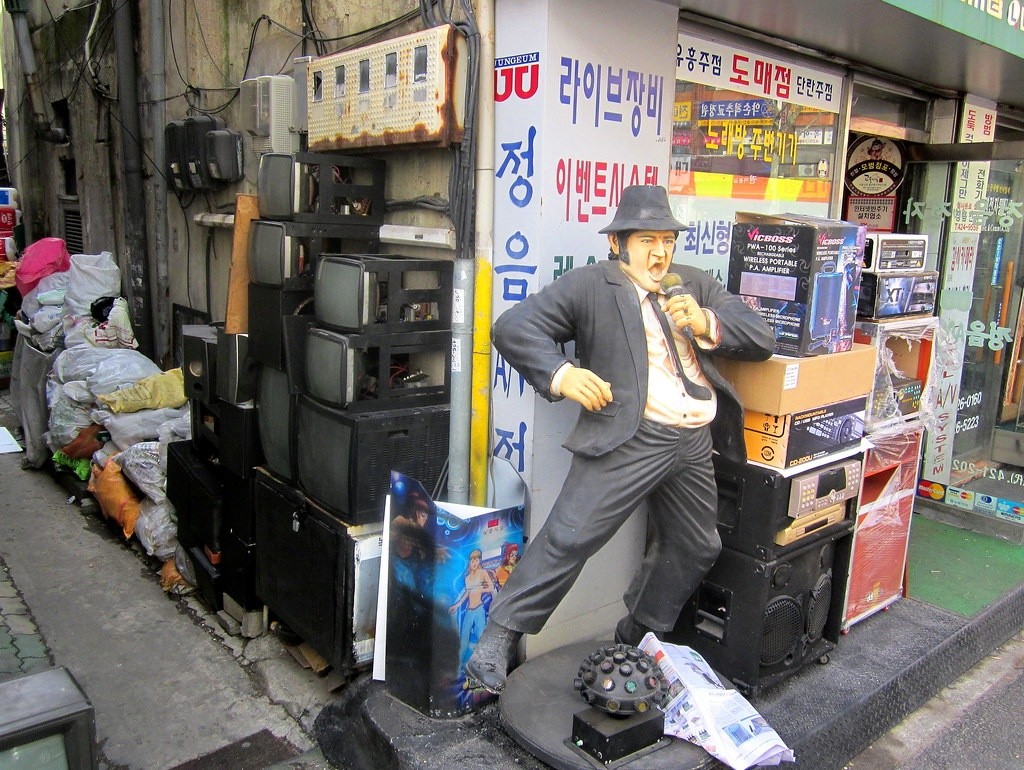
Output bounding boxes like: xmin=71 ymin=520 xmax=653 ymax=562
xmin=189 ymin=397 xmax=263 ymax=482
xmin=249 ymin=152 xmax=454 ymax=523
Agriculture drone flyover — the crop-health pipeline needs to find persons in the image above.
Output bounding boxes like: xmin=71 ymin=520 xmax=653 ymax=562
xmin=464 ymin=186 xmax=778 ymax=693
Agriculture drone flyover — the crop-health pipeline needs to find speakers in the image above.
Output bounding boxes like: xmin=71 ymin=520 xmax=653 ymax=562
xmin=663 ymin=525 xmax=855 ymax=696
xmin=182 ymin=332 xmax=258 ymax=411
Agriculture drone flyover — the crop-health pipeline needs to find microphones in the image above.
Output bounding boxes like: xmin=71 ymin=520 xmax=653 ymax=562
xmin=660 ymin=272 xmax=694 ymax=342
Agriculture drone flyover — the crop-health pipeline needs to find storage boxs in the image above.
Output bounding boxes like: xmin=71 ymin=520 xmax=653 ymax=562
xmin=726 ymin=210 xmax=866 ymax=357
xmin=710 ymin=343 xmax=876 ymax=468
xmin=384 ymin=470 xmax=524 ymax=720
xmin=857 ymin=271 xmax=939 ymax=320
xmin=855 ymin=315 xmax=939 ymax=433
xmin=862 ymin=233 xmax=929 ymax=274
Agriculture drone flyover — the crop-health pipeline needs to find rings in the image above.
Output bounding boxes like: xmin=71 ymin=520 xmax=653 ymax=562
xmin=683 ymin=308 xmax=689 ymax=315
xmin=686 ymin=317 xmax=691 ymax=323
xmin=682 ymin=301 xmax=688 ymax=307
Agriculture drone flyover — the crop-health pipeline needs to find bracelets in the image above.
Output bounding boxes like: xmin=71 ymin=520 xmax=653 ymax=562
xmin=700 ymin=309 xmax=711 ymax=338
